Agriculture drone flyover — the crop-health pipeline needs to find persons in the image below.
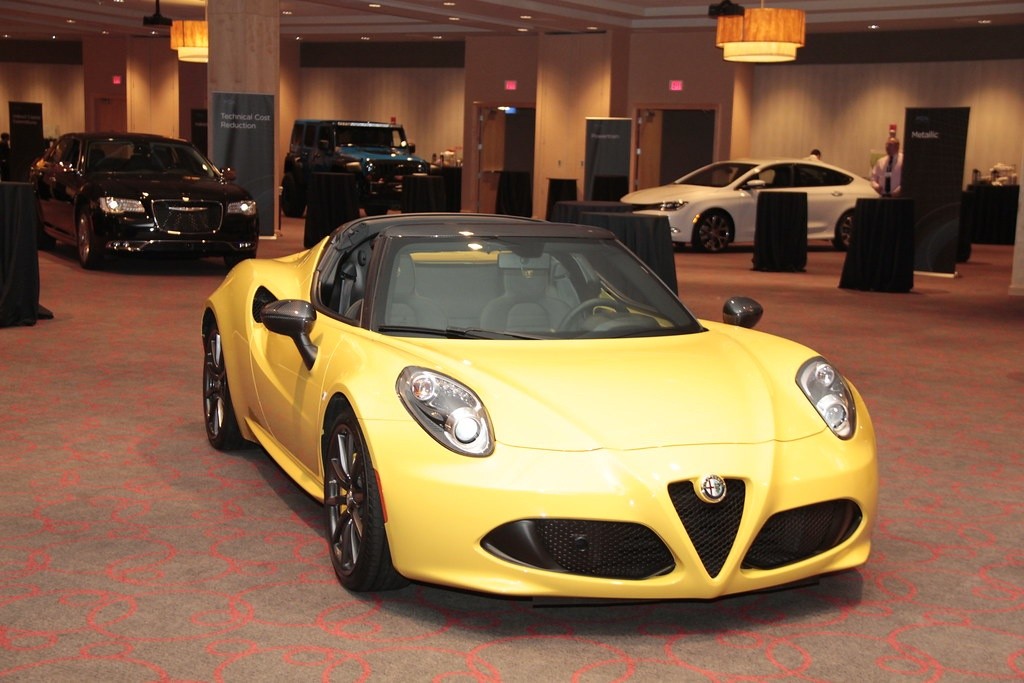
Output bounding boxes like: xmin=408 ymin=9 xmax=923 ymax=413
xmin=871 ymin=137 xmax=903 ymax=199
xmin=802 ymin=149 xmax=822 ymax=165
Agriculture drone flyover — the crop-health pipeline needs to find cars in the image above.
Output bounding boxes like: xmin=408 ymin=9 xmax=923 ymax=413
xmin=30 ymin=132 xmax=260 ymax=272
xmin=618 ymin=156 xmax=883 ymax=254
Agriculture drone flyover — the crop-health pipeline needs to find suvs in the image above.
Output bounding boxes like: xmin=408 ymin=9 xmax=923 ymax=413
xmin=277 ymin=119 xmax=433 ymax=218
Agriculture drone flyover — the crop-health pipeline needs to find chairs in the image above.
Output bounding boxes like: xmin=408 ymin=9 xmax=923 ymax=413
xmin=90 ymin=149 xmax=110 ymax=167
xmin=345 ymin=251 xmax=450 ymax=326
xmin=126 ymin=143 xmax=159 ymax=170
xmin=481 ymin=268 xmax=589 ymax=328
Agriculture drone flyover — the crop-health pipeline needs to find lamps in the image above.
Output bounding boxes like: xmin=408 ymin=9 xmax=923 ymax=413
xmin=716 ymin=0 xmax=806 ymax=65
xmin=167 ymin=0 xmax=209 ymax=64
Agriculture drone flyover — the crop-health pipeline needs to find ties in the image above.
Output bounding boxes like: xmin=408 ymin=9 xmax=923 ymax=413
xmin=884 ymin=156 xmax=892 ymax=193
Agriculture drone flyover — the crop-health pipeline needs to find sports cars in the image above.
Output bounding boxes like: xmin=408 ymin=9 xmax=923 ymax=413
xmin=201 ymin=211 xmax=881 ymax=604
